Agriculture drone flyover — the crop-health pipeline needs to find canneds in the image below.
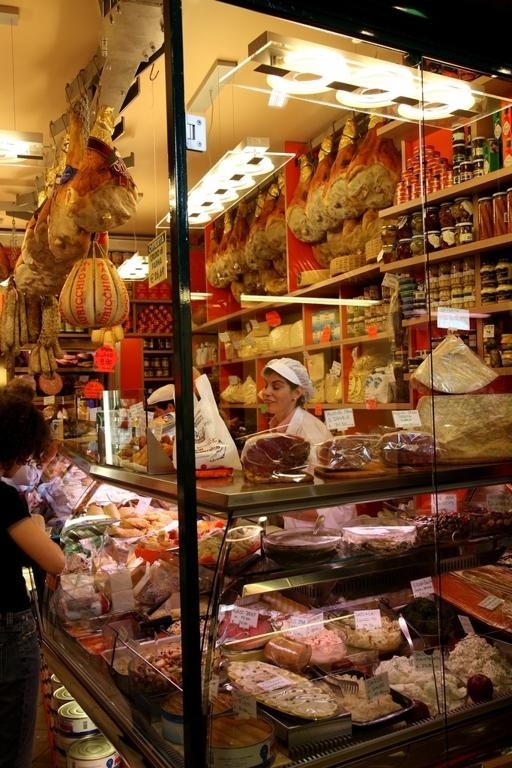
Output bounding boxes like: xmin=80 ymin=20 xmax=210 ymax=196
xmin=40 ymin=655 xmax=121 ymax=768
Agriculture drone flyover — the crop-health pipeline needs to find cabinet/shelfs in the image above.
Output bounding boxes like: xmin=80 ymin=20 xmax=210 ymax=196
xmin=29 ymin=435 xmax=511 ymax=768
xmin=12 ymin=65 xmax=512 ymax=453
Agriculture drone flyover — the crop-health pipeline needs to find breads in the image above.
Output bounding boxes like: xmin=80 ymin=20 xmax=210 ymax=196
xmin=107 ymin=508 xmax=171 ymax=539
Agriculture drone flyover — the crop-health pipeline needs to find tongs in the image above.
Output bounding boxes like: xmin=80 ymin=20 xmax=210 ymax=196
xmin=302 ymin=664 xmax=360 ymax=698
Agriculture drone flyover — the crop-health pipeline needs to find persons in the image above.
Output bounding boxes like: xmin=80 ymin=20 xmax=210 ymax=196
xmin=260 ymin=357 xmax=361 ymax=531
xmin=147 ymin=383 xmax=176 ymax=417
xmin=0 ymin=376 xmax=65 ymax=767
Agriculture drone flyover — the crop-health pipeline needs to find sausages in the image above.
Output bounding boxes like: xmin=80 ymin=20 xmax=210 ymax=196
xmin=263 ymin=637 xmax=312 ymax=671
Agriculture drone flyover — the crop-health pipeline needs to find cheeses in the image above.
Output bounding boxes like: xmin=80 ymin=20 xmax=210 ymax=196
xmin=408 ymin=334 xmax=499 ymax=394
xmin=417 ymin=394 xmax=512 ymax=464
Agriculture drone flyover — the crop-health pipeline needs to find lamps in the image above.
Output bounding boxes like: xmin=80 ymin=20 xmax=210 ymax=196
xmin=214 ymin=26 xmax=512 ymax=133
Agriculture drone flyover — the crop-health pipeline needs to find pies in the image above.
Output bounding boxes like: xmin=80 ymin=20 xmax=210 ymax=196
xmin=227 ymin=660 xmax=338 ymax=720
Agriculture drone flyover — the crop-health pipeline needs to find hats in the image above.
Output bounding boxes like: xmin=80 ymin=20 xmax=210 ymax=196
xmin=145 ymin=382 xmax=176 ymax=404
xmin=261 ymin=356 xmax=313 ymax=393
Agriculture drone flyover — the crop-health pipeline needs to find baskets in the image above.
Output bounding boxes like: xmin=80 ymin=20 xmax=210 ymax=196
xmin=361 ymin=236 xmax=383 ymax=262
xmin=328 ymin=253 xmax=366 ymax=274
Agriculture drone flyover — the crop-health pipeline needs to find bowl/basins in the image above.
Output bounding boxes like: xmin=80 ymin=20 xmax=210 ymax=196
xmin=264 ymin=526 xmax=343 ymax=566
xmin=289 ymin=611 xmax=404 ymax=667
xmin=340 ymin=526 xmax=417 ymax=559
